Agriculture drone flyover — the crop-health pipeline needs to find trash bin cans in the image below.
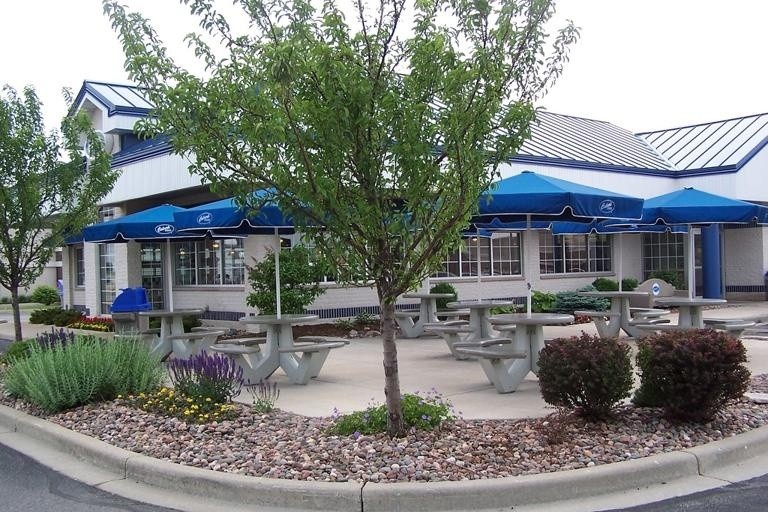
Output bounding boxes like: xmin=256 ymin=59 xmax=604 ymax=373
xmin=57 ymin=279 xmax=63 ymax=305
xmin=110 ymin=288 xmax=151 ymax=333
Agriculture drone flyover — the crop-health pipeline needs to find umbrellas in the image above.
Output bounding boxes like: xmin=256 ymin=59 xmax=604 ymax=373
xmin=386 ymin=211 xmax=492 ymax=293
xmin=406 ymin=170 xmax=644 ymax=318
xmin=173 ymin=188 xmax=341 ymax=319
xmin=603 ymin=186 xmax=768 ymax=299
xmin=62 ymin=203 xmax=249 ymax=311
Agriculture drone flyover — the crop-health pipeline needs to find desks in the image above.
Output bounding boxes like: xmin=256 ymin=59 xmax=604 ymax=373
xmin=446 ymin=300 xmax=514 ymax=360
xmin=577 ymin=291 xmax=650 ymax=340
xmin=402 ymin=293 xmax=454 ymax=339
xmin=486 ymin=313 xmax=575 ymax=393
xmin=138 ymin=308 xmax=320 ymax=385
xmin=655 ymin=298 xmax=727 ymax=330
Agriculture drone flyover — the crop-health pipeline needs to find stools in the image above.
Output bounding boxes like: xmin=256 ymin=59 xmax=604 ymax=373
xmin=714 ymin=320 xmax=756 ymax=332
xmin=393 ymin=307 xmax=757 ymax=393
xmin=451 ymin=337 xmax=512 ymax=349
xmin=701 ymin=316 xmax=744 ymax=324
xmin=455 ymin=347 xmax=528 ymax=361
xmin=113 ymin=326 xmax=350 ymax=386
